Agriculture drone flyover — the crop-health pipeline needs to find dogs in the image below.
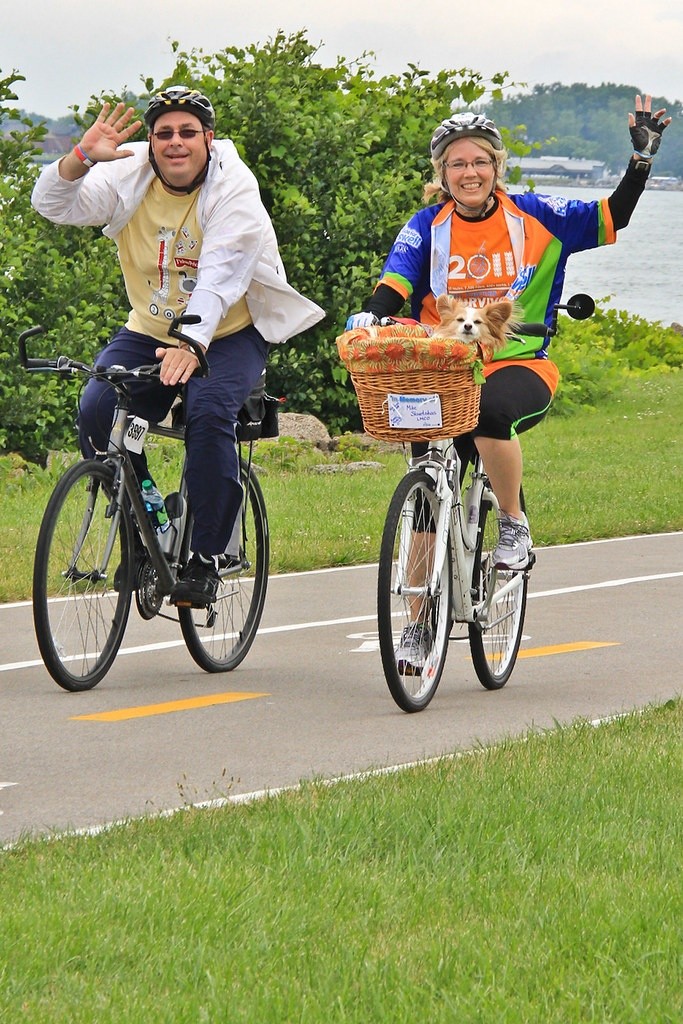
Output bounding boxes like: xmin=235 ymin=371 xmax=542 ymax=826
xmin=430 ymin=293 xmax=527 ymax=352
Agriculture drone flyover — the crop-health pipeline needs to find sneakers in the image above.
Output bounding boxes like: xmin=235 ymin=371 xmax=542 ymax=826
xmin=113 ymin=533 xmax=150 ymax=591
xmin=491 ymin=506 xmax=534 ymax=573
xmin=396 ymin=622 xmax=435 ymax=678
xmin=171 ymin=555 xmax=220 ymax=610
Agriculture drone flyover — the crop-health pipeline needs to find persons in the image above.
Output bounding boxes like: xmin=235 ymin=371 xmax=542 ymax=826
xmin=30 ymin=85 xmax=327 ymax=611
xmin=345 ymin=93 xmax=672 ymax=678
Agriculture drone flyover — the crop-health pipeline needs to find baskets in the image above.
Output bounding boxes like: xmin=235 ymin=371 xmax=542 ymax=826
xmin=334 ymin=321 xmax=484 ymax=442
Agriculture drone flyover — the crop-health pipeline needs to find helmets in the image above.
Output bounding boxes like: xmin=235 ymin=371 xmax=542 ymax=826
xmin=143 ymin=85 xmax=216 ymax=131
xmin=428 ymin=111 xmax=503 ymax=160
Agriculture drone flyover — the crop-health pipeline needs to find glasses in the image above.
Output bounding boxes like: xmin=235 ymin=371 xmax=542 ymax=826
xmin=151 ymin=127 xmax=209 ymax=141
xmin=443 ymin=158 xmax=503 ymax=172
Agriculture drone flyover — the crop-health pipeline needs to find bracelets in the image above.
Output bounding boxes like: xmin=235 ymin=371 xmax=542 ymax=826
xmin=73 ymin=143 xmax=98 ymax=168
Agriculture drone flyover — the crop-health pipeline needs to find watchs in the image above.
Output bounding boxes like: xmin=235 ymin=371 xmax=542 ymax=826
xmin=181 ymin=344 xmax=196 ymax=354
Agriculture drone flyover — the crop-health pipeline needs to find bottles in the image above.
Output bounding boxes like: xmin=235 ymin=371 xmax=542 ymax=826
xmin=140 ymin=480 xmax=170 ymax=535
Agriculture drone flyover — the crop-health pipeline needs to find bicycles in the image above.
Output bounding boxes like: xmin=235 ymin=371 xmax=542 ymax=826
xmin=340 ymin=292 xmax=596 ymax=714
xmin=15 ymin=314 xmax=279 ymax=694
